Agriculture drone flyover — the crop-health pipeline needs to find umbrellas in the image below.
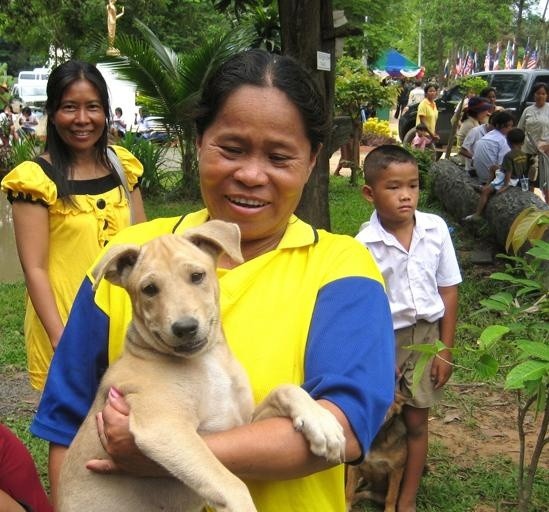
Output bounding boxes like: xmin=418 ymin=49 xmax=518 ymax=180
xmin=359 ymin=44 xmax=428 ymax=86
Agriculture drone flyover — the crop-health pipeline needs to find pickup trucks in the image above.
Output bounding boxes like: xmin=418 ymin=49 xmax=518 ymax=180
xmin=399 ymin=68 xmax=549 ymax=168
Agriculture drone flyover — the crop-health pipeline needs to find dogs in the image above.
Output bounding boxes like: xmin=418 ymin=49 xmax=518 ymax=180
xmin=55 ymin=218 xmax=347 ymax=512
xmin=344 ymin=364 xmax=432 ymax=512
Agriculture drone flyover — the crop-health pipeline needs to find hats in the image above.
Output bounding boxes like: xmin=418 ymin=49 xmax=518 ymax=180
xmin=463 ymin=97 xmax=494 ymax=111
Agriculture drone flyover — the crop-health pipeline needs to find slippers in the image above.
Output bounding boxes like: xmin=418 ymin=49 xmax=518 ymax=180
xmin=466 ymin=183 xmax=484 ymax=193
xmin=461 ymin=213 xmax=482 ymax=223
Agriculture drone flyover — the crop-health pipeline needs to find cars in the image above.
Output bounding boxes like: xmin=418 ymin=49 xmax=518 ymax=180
xmin=128 ymin=116 xmax=182 ymax=144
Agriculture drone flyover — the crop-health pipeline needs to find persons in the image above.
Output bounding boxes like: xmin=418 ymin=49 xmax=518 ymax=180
xmin=14 ymin=107 xmax=38 ymax=146
xmin=0 ymin=105 xmax=16 ymax=146
xmin=0 ymin=420 xmax=51 ymax=511
xmin=110 ymin=108 xmax=126 ymax=139
xmin=353 ymin=145 xmax=462 ymax=509
xmin=134 ymin=108 xmax=155 ymax=138
xmin=30 ymin=50 xmax=399 ymax=511
xmin=364 ymin=76 xmax=549 ymax=222
xmin=0 ymin=61 xmax=151 ymax=396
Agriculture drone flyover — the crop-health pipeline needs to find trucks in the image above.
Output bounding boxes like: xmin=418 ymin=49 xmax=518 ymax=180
xmin=17 ymin=68 xmax=54 ymax=113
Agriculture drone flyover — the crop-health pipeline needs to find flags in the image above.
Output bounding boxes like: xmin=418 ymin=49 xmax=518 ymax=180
xmin=443 ymin=38 xmax=548 ymax=81
